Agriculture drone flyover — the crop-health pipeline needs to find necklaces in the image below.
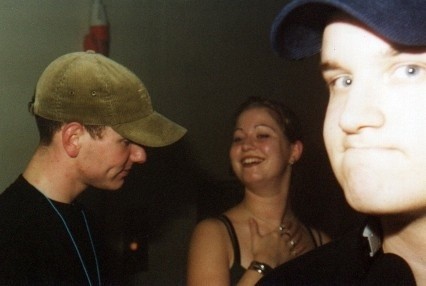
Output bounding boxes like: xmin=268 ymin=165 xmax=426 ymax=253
xmin=43 ymin=193 xmax=102 ymax=286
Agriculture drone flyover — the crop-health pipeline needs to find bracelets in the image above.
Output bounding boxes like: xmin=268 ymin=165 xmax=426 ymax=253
xmin=248 ymin=260 xmax=273 ymax=276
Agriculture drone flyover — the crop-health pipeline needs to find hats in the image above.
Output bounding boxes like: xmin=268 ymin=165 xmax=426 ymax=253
xmin=270 ymin=0 xmax=426 ymax=62
xmin=28 ymin=50 xmax=187 ymax=146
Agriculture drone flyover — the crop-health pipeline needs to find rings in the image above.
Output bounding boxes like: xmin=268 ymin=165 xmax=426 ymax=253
xmin=289 ymin=239 xmax=296 ymax=247
xmin=279 ymin=224 xmax=285 ymax=230
xmin=292 ymin=250 xmax=297 ymax=256
xmin=281 ymin=232 xmax=291 ymax=241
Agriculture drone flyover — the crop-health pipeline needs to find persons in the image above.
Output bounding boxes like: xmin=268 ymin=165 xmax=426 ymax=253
xmin=187 ymin=93 xmax=333 ymax=286
xmin=1 ymin=49 xmax=187 ymax=286
xmin=249 ymin=0 xmax=426 ymax=286
